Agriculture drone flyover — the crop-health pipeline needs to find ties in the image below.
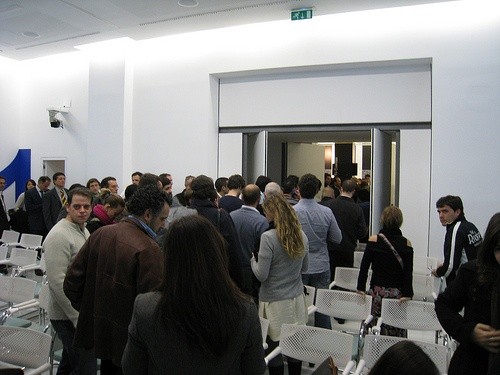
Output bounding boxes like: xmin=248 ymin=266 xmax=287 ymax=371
xmin=39 ymin=190 xmax=43 ymax=200
xmin=60 ymin=189 xmax=69 ymax=207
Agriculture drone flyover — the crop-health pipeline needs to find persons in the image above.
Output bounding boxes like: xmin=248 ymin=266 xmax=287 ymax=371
xmin=435 ymin=212 xmax=500 ymax=375
xmin=357 ymin=206 xmax=414 ymax=338
xmin=0 ymin=176 xmax=10 ymax=239
xmin=43 ymin=188 xmax=96 ymax=375
xmin=122 ymin=215 xmax=267 ymax=375
xmin=368 ymin=340 xmax=440 ymax=375
xmin=0 ymin=172 xmax=370 ymax=330
xmin=250 ymin=191 xmax=309 ymax=375
xmin=62 ymin=185 xmax=173 ymax=375
xmin=433 ymin=195 xmax=482 ymax=286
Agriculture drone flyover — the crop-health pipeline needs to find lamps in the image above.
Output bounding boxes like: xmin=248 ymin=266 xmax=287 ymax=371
xmin=48 ymin=110 xmax=63 ymax=129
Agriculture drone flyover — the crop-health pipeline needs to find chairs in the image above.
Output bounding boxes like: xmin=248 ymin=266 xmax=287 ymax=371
xmin=0 ymin=231 xmax=100 ymax=375
xmin=262 ymin=250 xmax=456 ymax=375
